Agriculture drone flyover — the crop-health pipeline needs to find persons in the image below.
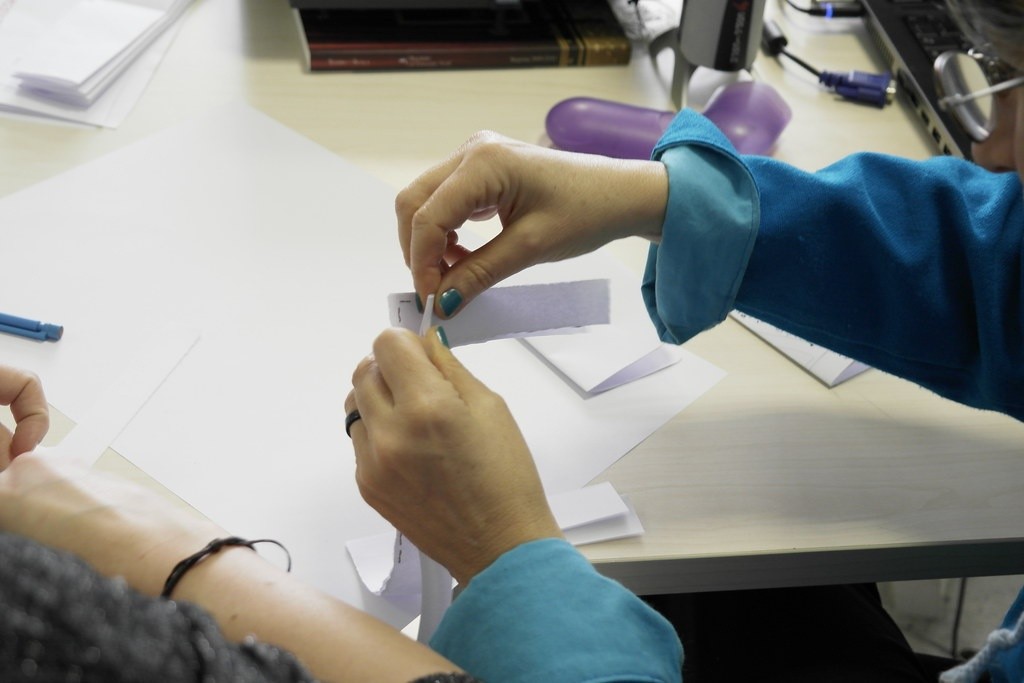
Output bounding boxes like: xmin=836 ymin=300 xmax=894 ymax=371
xmin=1 ymin=361 xmax=492 ymax=683
xmin=344 ymin=108 xmax=1024 ymax=683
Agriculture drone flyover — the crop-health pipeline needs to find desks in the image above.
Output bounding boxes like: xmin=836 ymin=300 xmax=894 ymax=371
xmin=0 ymin=0 xmax=1024 ymax=598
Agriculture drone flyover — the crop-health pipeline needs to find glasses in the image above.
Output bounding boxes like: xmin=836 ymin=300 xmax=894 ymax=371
xmin=933 ymin=43 xmax=1024 ymax=143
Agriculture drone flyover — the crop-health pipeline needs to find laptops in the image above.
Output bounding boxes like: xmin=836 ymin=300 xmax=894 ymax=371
xmin=858 ymin=0 xmax=1015 ymax=163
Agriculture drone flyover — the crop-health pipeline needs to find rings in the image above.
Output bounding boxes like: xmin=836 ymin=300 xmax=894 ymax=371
xmin=345 ymin=410 xmax=360 ymax=437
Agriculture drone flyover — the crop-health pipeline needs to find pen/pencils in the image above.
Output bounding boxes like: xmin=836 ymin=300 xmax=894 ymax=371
xmin=0 ymin=312 xmax=64 ymax=342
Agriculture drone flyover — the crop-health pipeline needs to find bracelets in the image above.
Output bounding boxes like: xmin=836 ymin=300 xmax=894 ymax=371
xmin=161 ymin=538 xmax=293 ymax=602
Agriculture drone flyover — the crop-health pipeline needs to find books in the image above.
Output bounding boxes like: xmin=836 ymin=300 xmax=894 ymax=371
xmin=285 ymin=0 xmax=633 ymax=74
